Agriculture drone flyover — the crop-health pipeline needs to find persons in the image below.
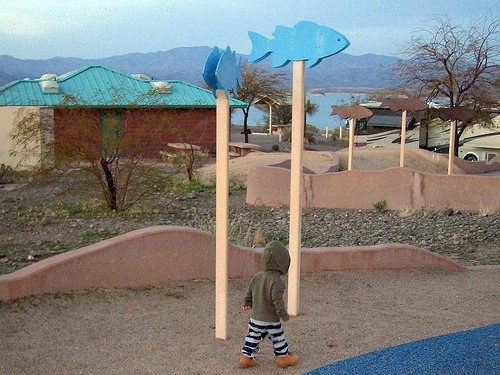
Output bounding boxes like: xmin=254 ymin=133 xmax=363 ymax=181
xmin=239 ymin=241 xmax=299 ymax=369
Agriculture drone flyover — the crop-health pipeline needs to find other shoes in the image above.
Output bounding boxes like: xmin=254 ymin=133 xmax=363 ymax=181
xmin=276 ymin=351 xmax=299 ymax=367
xmin=240 ymin=354 xmax=257 ymax=367
